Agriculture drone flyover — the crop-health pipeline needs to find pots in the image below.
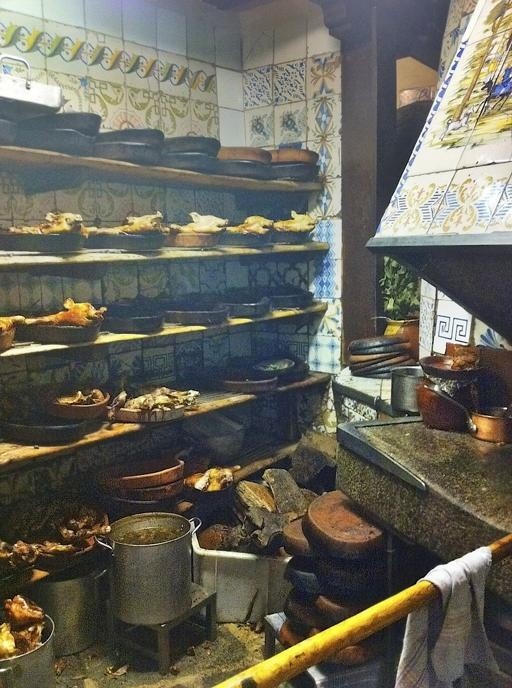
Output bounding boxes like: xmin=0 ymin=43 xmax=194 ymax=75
xmin=31 ymin=560 xmax=109 ymax=656
xmin=93 ymin=511 xmax=202 ymax=626
xmin=424 ymin=384 xmax=512 ymax=443
xmin=0 ymin=608 xmax=56 ymax=687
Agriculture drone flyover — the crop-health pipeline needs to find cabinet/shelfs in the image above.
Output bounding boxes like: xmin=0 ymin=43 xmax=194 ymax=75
xmin=0 ymin=142 xmax=329 ymax=597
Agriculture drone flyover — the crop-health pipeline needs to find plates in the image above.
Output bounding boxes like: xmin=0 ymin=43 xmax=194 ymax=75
xmin=347 ymin=333 xmax=417 ymax=379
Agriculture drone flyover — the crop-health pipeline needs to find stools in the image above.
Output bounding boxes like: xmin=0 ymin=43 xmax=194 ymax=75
xmin=264 ymin=612 xmax=400 ymax=688
xmin=106 ymin=583 xmax=217 ymax=676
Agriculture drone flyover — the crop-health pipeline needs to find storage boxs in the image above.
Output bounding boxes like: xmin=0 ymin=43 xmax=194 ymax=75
xmin=190 ymin=514 xmax=295 ymax=624
